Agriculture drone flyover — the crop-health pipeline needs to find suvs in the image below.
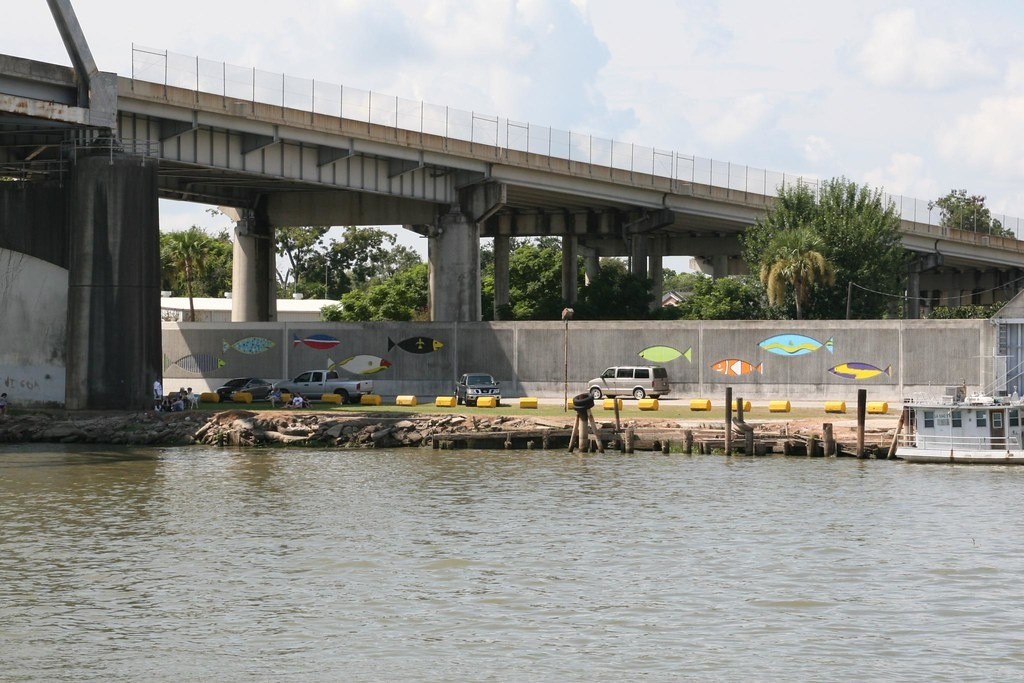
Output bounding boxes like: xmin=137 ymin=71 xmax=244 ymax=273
xmin=454 ymin=373 xmax=502 ymax=407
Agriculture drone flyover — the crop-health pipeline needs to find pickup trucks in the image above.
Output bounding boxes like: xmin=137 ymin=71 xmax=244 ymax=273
xmin=268 ymin=369 xmax=374 ymax=405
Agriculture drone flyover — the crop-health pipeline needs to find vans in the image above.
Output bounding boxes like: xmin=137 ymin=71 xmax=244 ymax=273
xmin=586 ymin=366 xmax=671 ymax=400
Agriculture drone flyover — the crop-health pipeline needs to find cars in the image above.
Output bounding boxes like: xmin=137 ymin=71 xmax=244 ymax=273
xmin=215 ymin=378 xmax=273 ymax=401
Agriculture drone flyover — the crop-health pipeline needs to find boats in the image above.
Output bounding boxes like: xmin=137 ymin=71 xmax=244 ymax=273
xmin=893 ymin=379 xmax=1024 ymax=464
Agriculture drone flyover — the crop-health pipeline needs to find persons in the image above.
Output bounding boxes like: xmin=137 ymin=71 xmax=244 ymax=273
xmin=153 ymin=375 xmax=198 ymax=412
xmin=267 ymin=386 xmax=313 ymax=410
